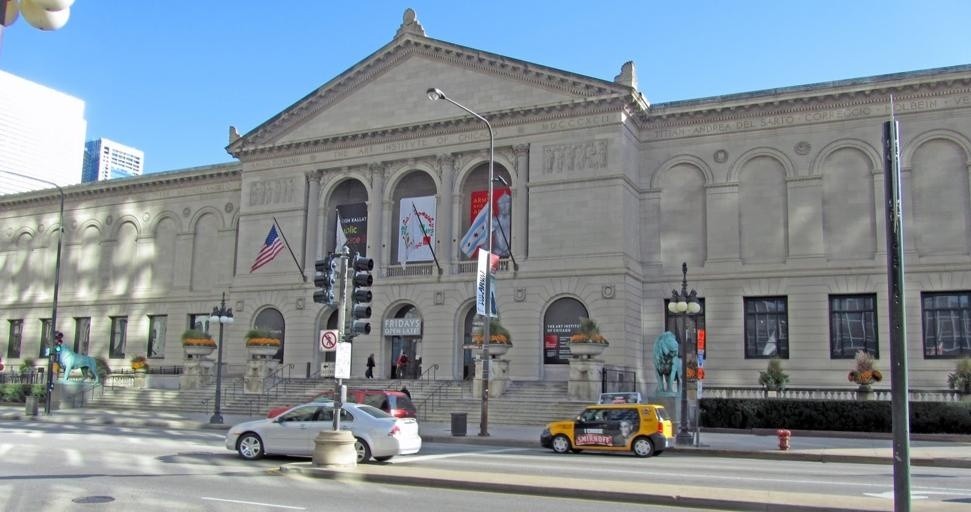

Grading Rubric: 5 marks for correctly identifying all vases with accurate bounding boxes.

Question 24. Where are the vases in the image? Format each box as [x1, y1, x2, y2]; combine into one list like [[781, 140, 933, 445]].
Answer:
[[475, 343, 512, 360], [247, 343, 279, 357], [569, 340, 606, 357], [851, 384, 880, 404], [182, 344, 220, 357]]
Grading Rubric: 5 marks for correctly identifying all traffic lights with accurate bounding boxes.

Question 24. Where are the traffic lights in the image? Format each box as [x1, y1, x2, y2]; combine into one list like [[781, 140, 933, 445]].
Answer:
[[311, 257, 330, 303], [354, 257, 376, 337], [51, 332, 63, 353]]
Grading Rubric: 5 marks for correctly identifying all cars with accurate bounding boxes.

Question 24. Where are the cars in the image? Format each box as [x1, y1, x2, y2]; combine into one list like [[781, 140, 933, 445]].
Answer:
[[224, 402, 423, 463]]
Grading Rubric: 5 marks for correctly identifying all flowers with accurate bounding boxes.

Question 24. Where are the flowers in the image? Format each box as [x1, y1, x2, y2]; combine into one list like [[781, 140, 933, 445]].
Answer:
[[843, 349, 882, 387], [468, 321, 511, 346], [562, 313, 609, 343], [242, 329, 285, 345], [177, 321, 220, 343]]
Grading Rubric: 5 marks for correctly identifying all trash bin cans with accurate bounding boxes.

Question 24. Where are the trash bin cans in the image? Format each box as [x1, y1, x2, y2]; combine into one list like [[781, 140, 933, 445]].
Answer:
[[449, 413, 467, 436], [24, 396, 38, 416]]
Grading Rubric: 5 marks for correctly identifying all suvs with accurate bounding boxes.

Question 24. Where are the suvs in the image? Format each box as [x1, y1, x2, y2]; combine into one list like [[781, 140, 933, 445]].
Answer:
[[540, 392, 677, 457], [268, 389, 417, 422]]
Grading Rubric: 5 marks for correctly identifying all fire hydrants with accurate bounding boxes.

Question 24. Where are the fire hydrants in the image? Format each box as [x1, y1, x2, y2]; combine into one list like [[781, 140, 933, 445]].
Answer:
[[778, 429, 793, 451]]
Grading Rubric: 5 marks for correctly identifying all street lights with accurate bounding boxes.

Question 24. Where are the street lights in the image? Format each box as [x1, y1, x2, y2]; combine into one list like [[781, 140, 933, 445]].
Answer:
[[669, 262, 703, 447], [209, 290, 233, 423], [425, 87, 495, 437]]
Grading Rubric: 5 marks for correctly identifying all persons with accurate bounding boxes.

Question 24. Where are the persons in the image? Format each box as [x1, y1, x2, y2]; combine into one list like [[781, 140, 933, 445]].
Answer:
[[367, 354, 376, 378], [397, 351, 411, 378]]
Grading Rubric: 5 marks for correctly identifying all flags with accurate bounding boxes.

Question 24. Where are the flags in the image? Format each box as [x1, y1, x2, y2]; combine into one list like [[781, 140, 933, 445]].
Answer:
[[459, 202, 498, 259], [249, 222, 285, 275], [334, 215, 349, 256], [400, 204, 426, 271]]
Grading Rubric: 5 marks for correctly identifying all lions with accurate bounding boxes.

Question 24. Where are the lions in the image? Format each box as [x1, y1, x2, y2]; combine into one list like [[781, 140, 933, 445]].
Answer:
[[652, 331, 683, 393], [55, 344, 100, 384]]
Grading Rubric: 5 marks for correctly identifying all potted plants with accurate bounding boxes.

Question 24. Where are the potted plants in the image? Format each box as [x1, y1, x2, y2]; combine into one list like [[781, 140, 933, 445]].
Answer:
[[941, 358, 971, 402]]
